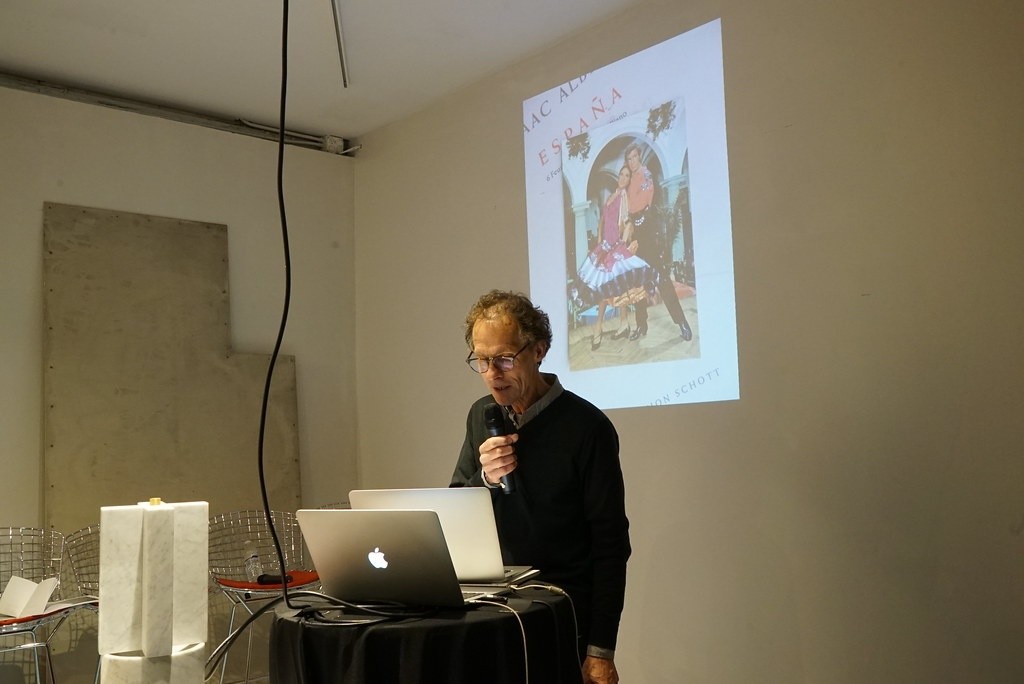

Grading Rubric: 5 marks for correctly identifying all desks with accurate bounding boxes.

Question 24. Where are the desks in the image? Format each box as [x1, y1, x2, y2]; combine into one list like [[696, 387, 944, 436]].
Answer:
[[269, 580, 579, 684]]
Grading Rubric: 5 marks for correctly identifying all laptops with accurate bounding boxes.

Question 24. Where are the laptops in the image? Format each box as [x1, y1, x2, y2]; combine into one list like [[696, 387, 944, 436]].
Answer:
[[348, 487, 534, 584], [296, 508, 518, 614]]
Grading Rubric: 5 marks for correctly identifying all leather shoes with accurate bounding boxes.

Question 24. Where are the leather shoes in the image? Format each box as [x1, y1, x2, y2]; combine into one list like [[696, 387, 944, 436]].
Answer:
[[630, 323, 648, 340], [679, 320, 692, 341]]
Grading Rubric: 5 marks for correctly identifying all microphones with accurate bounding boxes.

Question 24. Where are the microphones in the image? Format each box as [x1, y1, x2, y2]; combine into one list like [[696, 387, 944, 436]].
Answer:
[[257, 574, 293, 585], [482, 403, 515, 496]]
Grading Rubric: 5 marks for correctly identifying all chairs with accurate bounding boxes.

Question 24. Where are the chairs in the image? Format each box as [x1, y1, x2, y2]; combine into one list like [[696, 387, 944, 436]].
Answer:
[[0, 526, 70, 684], [209, 509, 321, 684], [65, 523, 102, 684]]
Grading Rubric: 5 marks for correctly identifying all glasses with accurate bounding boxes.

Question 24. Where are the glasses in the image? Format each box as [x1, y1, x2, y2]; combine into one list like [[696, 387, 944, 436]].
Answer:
[[466, 339, 533, 373]]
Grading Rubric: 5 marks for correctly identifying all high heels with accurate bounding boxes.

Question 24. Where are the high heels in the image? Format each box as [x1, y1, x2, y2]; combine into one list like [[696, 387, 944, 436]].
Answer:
[[611, 328, 630, 340], [591, 331, 602, 351]]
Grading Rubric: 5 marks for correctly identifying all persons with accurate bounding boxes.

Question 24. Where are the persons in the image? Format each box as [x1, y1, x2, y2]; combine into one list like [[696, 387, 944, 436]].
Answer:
[[577, 165, 660, 352], [448, 289, 632, 684], [625, 143, 693, 341]]
